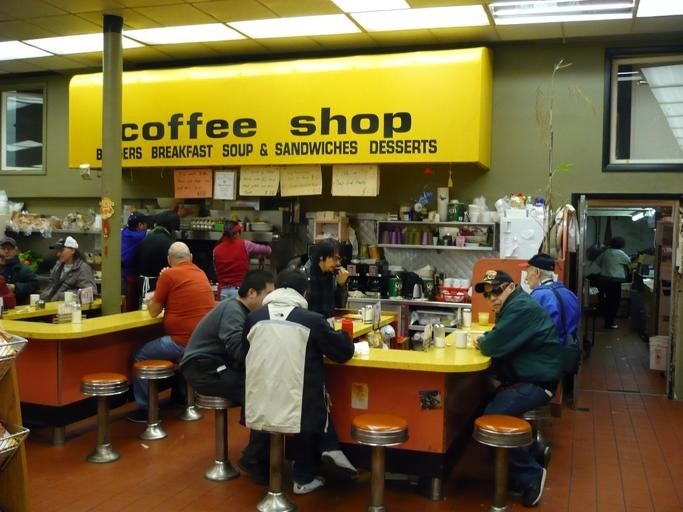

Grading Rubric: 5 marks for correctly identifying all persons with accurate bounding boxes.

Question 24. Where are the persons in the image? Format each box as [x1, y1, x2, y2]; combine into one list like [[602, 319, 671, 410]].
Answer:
[[596, 236, 632, 329], [2, 212, 96, 307], [180, 239, 355, 494], [474, 254, 580, 506], [127, 242, 216, 423], [120, 214, 271, 301]]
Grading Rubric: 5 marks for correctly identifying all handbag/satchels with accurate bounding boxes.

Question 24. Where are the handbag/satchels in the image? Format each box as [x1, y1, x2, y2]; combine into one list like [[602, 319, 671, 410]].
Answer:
[[565, 335, 581, 374]]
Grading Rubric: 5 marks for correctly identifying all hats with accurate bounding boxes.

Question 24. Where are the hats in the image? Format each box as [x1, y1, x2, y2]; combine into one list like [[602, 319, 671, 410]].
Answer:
[[128, 212, 153, 225], [49, 235, 79, 250], [474, 269, 514, 292], [0, 237, 16, 247], [518, 253, 555, 271]]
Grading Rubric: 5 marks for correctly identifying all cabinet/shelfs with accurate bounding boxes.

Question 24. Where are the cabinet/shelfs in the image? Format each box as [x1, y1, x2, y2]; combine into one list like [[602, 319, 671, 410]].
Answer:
[[376, 220, 499, 251], [313, 217, 350, 244]]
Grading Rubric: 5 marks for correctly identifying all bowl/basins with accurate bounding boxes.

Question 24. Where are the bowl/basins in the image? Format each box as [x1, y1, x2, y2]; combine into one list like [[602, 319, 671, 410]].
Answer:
[[157, 198, 184, 209], [444, 292, 466, 303]]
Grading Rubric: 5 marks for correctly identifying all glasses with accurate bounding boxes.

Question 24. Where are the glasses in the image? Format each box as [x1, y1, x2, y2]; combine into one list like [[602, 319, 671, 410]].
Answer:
[[483, 283, 511, 299]]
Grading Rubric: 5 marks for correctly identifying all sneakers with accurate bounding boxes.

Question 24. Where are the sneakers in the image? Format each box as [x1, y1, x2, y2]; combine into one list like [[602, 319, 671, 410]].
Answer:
[[534, 441, 551, 467], [159, 400, 184, 409], [124, 408, 164, 423], [293, 475, 326, 494], [522, 467, 547, 507], [605, 322, 619, 329], [321, 450, 360, 482], [237, 454, 269, 485]]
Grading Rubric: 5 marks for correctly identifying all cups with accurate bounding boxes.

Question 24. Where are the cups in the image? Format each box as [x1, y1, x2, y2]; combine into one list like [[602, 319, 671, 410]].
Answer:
[[463, 308, 472, 326], [478, 312, 490, 326], [469, 204, 496, 222], [381, 230, 438, 245], [454, 331, 479, 349], [357, 304, 372, 325]]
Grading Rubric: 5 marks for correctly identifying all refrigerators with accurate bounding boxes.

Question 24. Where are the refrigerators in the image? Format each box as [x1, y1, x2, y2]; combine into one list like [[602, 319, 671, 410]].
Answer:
[[500, 216, 545, 259]]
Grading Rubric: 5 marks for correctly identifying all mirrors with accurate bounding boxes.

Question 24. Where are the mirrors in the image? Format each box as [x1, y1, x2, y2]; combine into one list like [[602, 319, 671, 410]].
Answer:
[[601, 45, 683, 172], [0, 81, 48, 176]]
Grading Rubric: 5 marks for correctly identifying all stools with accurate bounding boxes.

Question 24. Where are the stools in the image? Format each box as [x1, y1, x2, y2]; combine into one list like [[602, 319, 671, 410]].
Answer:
[[473, 413, 534, 512], [350, 413, 410, 512], [254, 428, 298, 512], [518, 407, 547, 471]]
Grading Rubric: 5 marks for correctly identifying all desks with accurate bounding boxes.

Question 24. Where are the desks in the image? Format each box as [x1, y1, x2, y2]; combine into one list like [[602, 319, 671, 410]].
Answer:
[[0, 295, 497, 502]]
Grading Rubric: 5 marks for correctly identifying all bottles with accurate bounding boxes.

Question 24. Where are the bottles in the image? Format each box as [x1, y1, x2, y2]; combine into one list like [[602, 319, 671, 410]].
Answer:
[[404, 211, 411, 221], [412, 283, 421, 298], [525, 195, 545, 218], [72, 304, 82, 324], [192, 217, 225, 232], [433, 323, 446, 347], [244, 219, 251, 232], [342, 318, 353, 340], [35, 299, 45, 309]]
